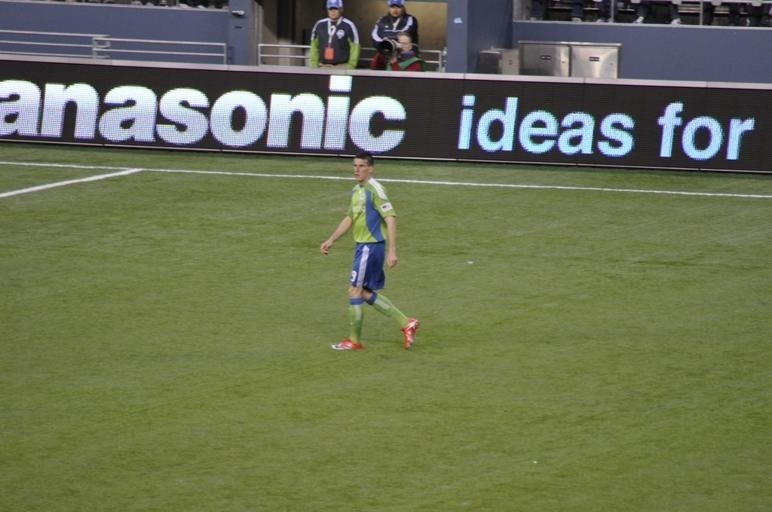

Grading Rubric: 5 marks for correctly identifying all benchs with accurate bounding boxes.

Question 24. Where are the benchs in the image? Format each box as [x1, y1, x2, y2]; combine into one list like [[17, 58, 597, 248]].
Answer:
[[546, 0, 772, 27]]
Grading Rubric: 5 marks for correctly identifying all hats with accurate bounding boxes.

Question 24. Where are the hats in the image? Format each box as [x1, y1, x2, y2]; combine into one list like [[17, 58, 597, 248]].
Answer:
[[326, 0, 343, 9], [387, 0, 405, 7]]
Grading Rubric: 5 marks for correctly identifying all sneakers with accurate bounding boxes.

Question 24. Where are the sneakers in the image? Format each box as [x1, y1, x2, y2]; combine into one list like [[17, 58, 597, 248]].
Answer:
[[401, 318, 419, 349], [331, 339, 362, 350]]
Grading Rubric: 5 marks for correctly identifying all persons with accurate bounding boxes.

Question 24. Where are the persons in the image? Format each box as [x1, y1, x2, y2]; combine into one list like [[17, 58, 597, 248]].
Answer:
[[371, 0, 419, 54], [321, 151, 419, 350], [370, 33, 424, 72], [307, 0, 361, 70], [526, 2, 770, 28]]
